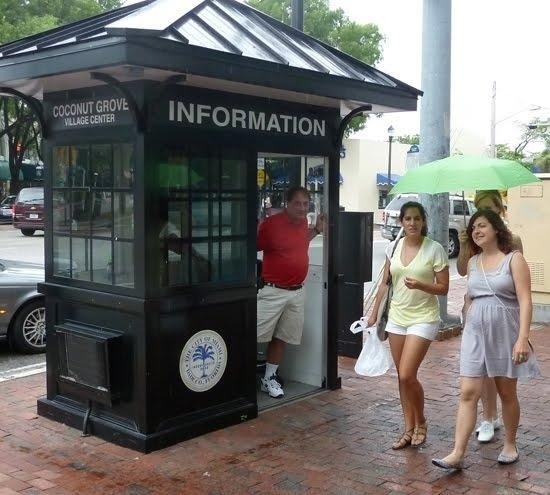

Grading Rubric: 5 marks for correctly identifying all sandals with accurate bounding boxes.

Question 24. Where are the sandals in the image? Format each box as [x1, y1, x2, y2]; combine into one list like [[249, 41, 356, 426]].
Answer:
[[409, 419, 428, 449], [390, 430, 414, 452]]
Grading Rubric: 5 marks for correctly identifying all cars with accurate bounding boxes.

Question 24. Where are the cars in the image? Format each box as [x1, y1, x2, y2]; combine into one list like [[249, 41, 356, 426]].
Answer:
[[1, 193, 17, 219], [0, 256, 81, 353]]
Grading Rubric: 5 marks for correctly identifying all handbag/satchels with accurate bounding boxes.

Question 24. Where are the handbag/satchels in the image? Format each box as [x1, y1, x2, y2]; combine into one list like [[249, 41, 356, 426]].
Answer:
[[375, 308, 392, 342]]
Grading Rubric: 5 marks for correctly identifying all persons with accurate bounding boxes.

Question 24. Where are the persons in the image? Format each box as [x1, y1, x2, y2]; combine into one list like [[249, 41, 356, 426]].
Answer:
[[366, 202, 449, 449], [430, 210, 532, 470], [457, 189, 524, 443], [262, 192, 273, 217], [256, 187, 324, 399]]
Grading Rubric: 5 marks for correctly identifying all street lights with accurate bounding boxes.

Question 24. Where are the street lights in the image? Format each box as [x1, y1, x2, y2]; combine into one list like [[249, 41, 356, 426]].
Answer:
[[385, 124, 396, 197]]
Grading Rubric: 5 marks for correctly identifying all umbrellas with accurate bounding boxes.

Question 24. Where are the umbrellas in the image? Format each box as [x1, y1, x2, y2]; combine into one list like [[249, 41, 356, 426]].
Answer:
[[388, 153, 542, 228], [159, 163, 203, 187]]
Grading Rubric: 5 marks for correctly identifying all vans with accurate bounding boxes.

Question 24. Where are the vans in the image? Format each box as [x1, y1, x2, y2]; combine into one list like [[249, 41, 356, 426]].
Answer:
[[12, 187, 72, 238]]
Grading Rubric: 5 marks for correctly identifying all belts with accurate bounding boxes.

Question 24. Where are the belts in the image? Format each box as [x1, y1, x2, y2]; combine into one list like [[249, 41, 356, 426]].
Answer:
[[262, 281, 305, 291]]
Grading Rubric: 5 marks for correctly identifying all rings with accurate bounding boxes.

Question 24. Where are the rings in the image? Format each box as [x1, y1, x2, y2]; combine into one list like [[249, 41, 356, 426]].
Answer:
[[518, 354, 524, 361]]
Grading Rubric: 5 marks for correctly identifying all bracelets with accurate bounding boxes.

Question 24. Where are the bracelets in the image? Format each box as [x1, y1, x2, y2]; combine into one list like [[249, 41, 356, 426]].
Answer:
[[314, 228, 320, 235]]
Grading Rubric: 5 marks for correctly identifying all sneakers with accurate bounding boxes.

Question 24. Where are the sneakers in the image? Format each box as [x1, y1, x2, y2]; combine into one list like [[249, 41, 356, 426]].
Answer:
[[430, 453, 465, 473], [259, 377, 285, 400], [474, 418, 503, 435], [496, 444, 520, 466], [476, 420, 496, 445]]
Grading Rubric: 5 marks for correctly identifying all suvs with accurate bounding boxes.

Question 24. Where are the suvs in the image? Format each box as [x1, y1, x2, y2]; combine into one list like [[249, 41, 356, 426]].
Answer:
[[381, 192, 482, 258]]
